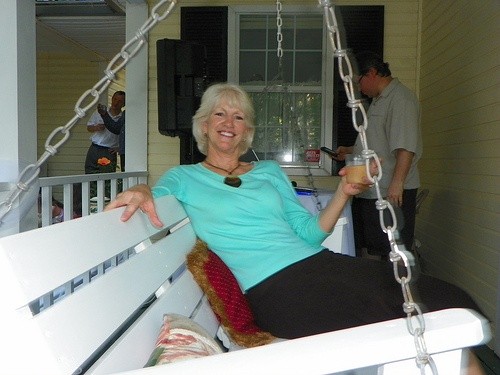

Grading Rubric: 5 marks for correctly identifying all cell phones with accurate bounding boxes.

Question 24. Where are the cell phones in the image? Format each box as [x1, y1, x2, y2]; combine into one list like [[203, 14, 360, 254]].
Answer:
[[320, 146, 337, 157], [101, 105, 106, 112]]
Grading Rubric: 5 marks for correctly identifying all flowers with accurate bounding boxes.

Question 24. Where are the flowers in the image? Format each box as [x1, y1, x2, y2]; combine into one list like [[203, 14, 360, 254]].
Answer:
[[90, 156, 120, 197]]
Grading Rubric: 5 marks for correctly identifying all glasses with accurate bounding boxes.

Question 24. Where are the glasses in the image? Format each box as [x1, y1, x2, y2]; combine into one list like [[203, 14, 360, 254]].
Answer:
[[353, 70, 370, 88]]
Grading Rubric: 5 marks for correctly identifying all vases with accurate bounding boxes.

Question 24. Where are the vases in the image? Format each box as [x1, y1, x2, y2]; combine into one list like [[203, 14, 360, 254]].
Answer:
[[90, 197, 110, 213]]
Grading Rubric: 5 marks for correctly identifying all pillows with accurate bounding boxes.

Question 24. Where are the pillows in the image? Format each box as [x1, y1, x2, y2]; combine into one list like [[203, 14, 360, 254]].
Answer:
[[148, 314, 226, 366], [186, 239, 271, 349]]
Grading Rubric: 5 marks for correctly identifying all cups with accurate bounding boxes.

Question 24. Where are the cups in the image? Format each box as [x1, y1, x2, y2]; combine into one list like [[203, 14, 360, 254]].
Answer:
[[344, 153, 372, 184]]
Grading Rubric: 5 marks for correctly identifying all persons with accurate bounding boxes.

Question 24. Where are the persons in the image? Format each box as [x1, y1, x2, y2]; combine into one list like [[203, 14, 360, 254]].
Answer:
[[84, 90, 125, 174], [103, 83, 492, 374], [329, 51, 424, 266], [97, 103, 125, 172]]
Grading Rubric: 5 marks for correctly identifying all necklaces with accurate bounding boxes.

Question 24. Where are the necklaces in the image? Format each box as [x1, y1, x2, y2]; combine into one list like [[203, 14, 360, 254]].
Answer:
[[199, 153, 254, 189]]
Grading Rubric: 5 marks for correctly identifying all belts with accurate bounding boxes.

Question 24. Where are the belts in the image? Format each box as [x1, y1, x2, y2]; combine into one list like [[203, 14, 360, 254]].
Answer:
[[92, 143, 109, 150]]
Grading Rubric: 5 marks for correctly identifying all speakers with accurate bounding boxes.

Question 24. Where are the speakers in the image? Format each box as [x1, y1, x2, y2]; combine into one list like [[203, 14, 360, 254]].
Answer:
[[156, 38, 207, 138]]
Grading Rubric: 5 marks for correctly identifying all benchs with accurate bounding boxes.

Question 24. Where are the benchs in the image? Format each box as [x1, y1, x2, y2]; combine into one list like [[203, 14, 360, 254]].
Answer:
[[0, 194, 494, 375]]
